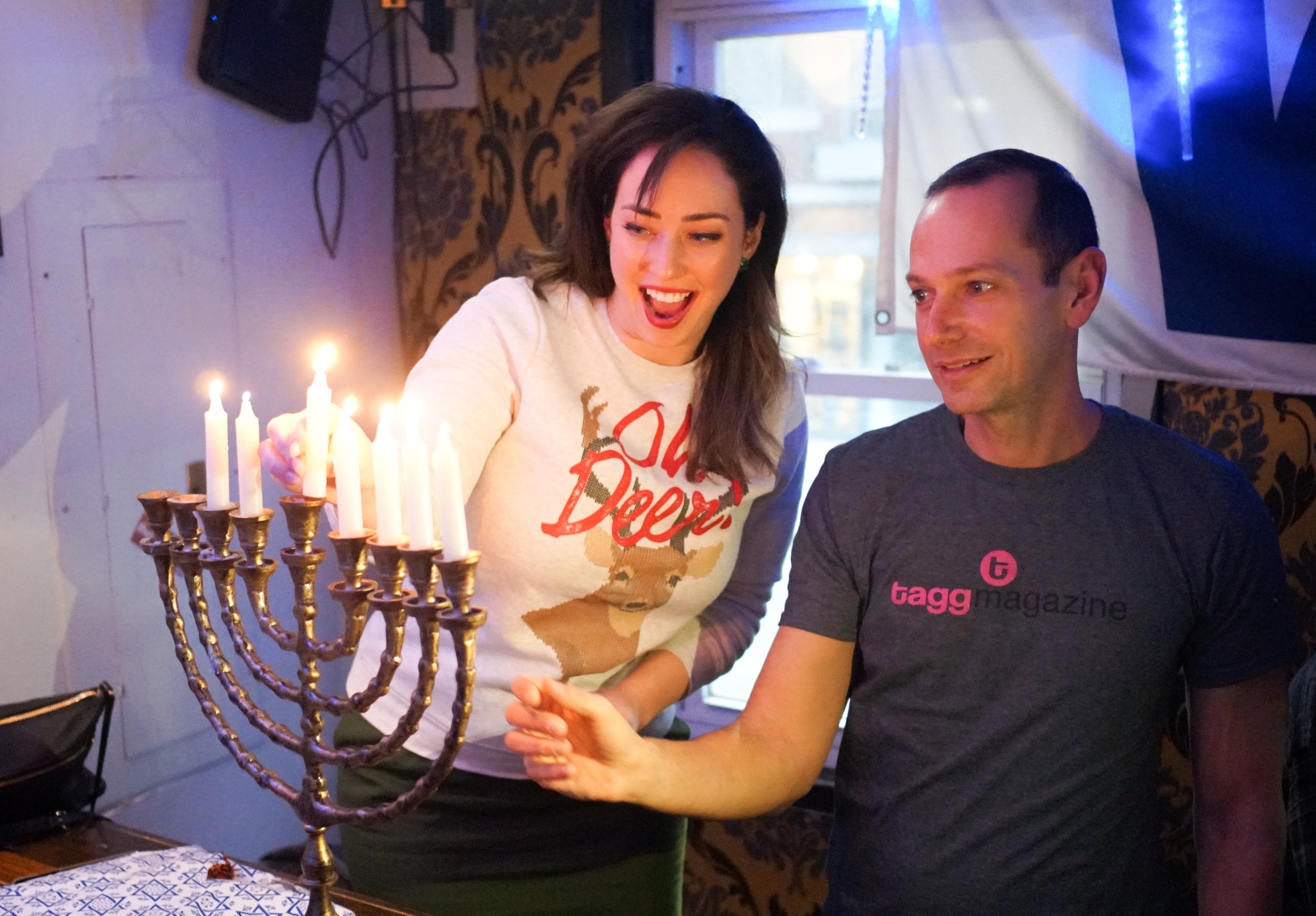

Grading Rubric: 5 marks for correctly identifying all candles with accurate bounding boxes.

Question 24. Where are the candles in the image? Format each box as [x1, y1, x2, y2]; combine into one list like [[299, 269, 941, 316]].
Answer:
[[203, 337, 469, 563]]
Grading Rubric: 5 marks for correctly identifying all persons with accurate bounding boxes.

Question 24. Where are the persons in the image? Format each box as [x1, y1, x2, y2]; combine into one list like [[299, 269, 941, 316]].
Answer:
[[505, 148, 1286, 916], [257, 84, 808, 915]]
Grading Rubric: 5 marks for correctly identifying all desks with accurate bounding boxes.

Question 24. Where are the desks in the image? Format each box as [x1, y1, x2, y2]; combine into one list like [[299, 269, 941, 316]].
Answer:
[[1, 813, 435, 916]]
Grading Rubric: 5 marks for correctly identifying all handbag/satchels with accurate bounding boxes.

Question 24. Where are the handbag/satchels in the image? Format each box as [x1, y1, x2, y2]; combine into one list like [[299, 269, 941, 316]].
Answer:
[[0, 681, 115, 851]]
